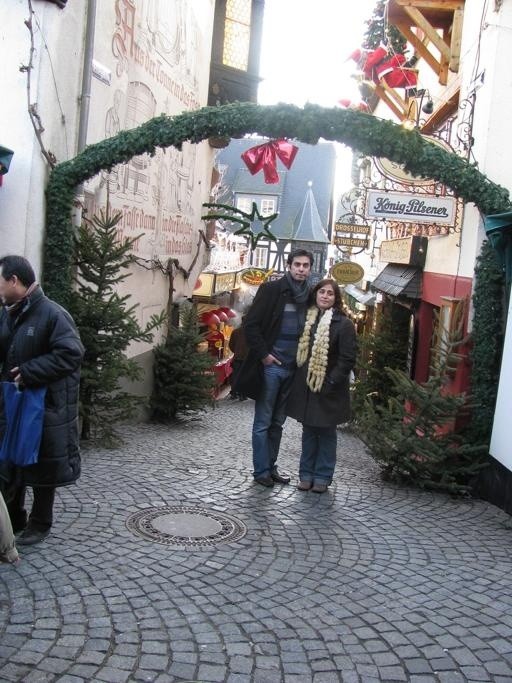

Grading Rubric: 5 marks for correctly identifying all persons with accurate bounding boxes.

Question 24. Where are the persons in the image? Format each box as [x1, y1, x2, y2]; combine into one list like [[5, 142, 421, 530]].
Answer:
[[244, 247, 315, 487], [283, 279, 356, 493], [229, 313, 251, 400], [1, 254, 86, 544], [0, 489, 19, 563]]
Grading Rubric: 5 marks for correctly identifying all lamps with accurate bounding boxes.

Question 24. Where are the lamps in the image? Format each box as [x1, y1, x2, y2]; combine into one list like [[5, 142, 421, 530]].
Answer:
[[404, 87, 434, 131]]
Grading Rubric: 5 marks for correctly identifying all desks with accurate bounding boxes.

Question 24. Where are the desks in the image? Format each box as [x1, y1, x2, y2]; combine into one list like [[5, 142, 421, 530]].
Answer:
[[209, 352, 235, 385]]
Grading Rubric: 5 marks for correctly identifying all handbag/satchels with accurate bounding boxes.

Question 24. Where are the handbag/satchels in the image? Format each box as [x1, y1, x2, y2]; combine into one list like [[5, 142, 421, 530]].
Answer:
[[1, 380, 47, 465]]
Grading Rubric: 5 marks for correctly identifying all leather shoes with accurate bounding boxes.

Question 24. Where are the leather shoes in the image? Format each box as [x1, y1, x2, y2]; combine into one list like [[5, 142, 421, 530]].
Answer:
[[15, 525, 52, 545], [255, 469, 328, 495]]
